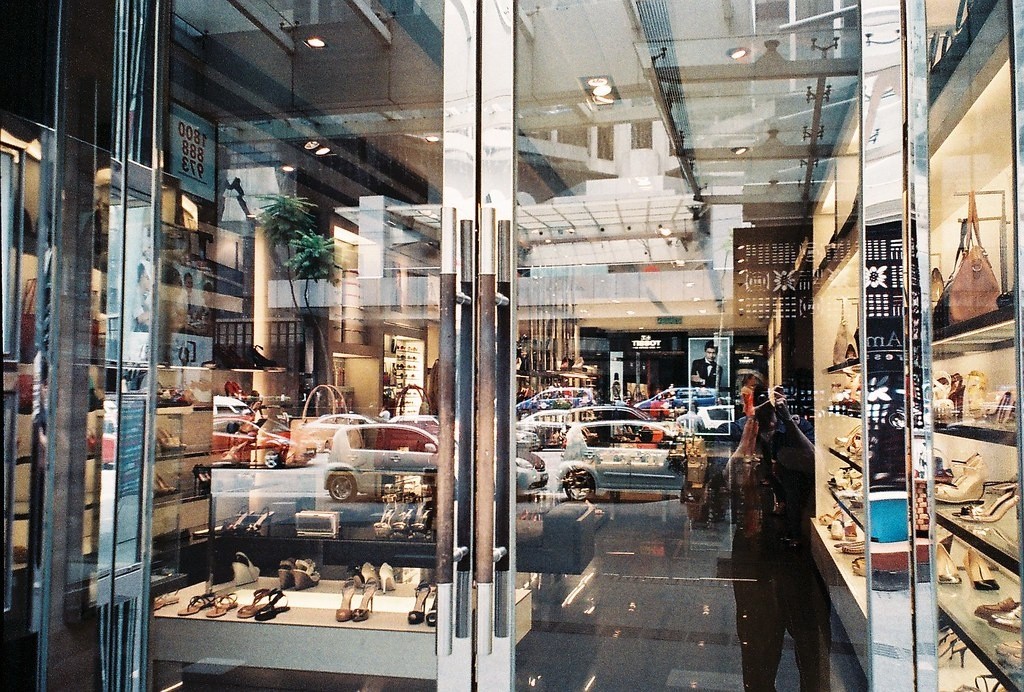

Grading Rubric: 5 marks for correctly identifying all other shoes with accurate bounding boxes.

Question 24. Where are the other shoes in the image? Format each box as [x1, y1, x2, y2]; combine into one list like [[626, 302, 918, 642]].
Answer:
[[214, 344, 277, 369]]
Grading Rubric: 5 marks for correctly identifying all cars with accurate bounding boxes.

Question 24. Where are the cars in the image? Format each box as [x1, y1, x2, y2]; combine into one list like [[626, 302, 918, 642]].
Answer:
[[210, 413, 313, 470], [323, 423, 547, 504], [316, 384, 747, 445], [561, 419, 695, 506]]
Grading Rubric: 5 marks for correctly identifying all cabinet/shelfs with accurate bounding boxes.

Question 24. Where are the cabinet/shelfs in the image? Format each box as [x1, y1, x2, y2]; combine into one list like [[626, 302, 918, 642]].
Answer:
[[805, 3, 1024, 692], [7, 136, 248, 616]]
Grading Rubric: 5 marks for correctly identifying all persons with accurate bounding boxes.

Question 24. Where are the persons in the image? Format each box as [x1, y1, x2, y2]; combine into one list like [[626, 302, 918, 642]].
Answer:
[[677, 405, 708, 433], [691, 339, 724, 405], [713, 360, 833, 692]]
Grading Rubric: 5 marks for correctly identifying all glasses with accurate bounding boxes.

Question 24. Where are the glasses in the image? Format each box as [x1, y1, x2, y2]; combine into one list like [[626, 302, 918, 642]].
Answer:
[[142, 275, 150, 280], [393, 346, 417, 416]]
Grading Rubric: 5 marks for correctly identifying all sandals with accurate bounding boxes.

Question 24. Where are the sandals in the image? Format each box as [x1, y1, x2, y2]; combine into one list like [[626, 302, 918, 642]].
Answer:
[[155, 589, 290, 621]]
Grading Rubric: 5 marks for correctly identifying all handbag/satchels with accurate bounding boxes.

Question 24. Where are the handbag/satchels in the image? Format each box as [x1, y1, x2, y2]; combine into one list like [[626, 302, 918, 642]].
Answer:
[[833, 191, 1000, 367], [20, 278, 38, 363], [382, 362, 389, 387], [19, 374, 33, 413], [286, 385, 363, 467], [374, 386, 440, 466]]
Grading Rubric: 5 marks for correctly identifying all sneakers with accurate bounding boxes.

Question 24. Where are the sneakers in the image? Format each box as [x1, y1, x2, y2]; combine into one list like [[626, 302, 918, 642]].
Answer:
[[743, 454, 761, 464]]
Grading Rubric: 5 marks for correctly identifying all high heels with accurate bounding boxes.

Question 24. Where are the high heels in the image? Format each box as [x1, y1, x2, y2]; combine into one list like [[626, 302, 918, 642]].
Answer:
[[221, 505, 274, 536], [278, 558, 321, 591], [154, 473, 179, 495], [819, 374, 1024, 692], [193, 464, 211, 488], [374, 502, 433, 539], [220, 382, 262, 462], [233, 552, 260, 586], [157, 427, 187, 450], [519, 510, 541, 521], [336, 562, 438, 628]]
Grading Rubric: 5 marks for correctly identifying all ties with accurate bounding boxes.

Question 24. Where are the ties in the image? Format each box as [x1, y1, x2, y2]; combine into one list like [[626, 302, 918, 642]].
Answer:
[[188, 295, 192, 310]]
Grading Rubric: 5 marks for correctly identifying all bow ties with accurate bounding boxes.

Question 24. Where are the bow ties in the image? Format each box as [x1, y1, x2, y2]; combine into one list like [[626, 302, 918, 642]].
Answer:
[[705, 363, 715, 367]]
